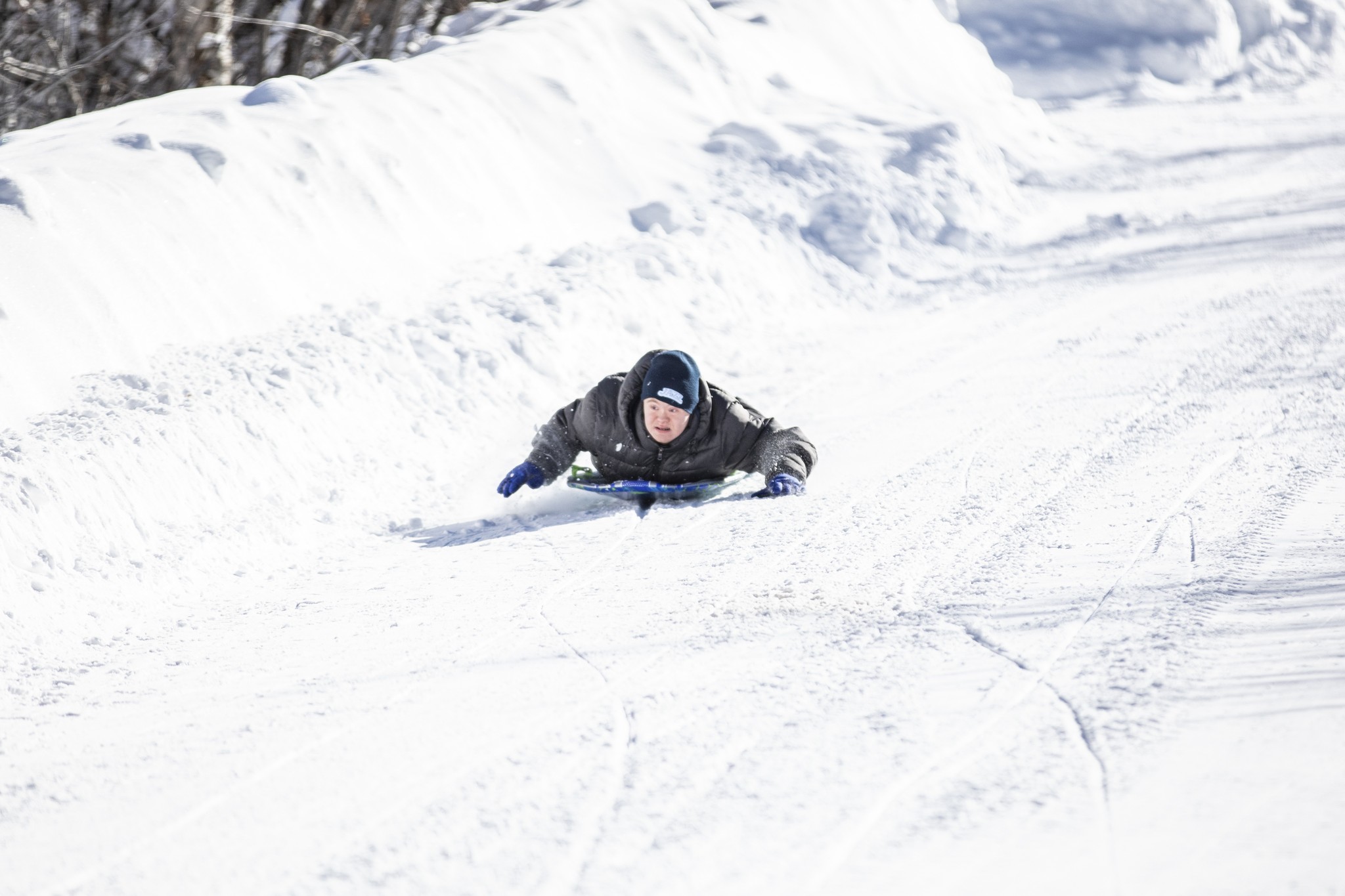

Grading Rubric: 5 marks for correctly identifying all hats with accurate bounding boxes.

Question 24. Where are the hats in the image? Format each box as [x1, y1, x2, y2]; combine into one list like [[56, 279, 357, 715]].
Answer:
[[641, 350, 701, 414]]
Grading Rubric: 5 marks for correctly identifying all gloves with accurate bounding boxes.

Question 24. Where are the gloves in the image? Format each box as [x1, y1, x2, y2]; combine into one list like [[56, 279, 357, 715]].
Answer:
[[751, 473, 807, 497], [496, 462, 544, 497]]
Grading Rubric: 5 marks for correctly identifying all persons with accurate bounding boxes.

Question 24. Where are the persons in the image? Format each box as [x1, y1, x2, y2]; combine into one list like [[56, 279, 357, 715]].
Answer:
[[496, 348, 820, 500]]
